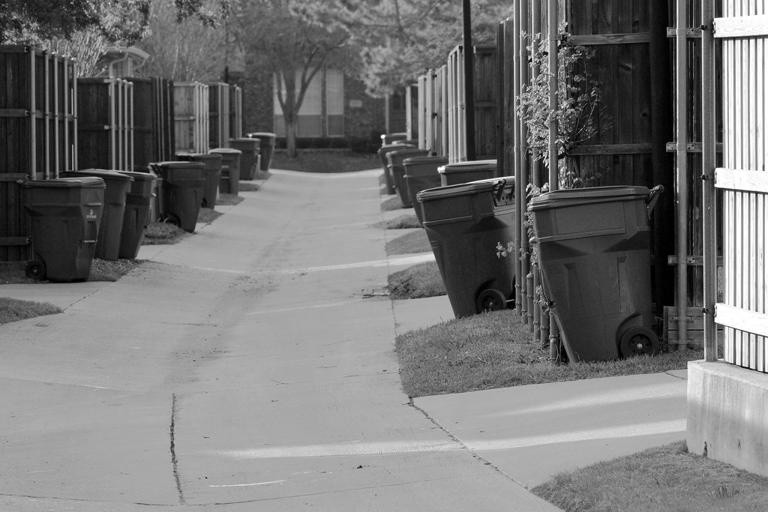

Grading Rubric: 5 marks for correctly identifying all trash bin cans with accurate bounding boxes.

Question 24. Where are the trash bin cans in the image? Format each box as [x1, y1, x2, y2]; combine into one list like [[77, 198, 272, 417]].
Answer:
[[377, 132, 498, 228], [526, 184, 665, 366], [22, 132, 276, 282], [416, 176, 515, 318]]
